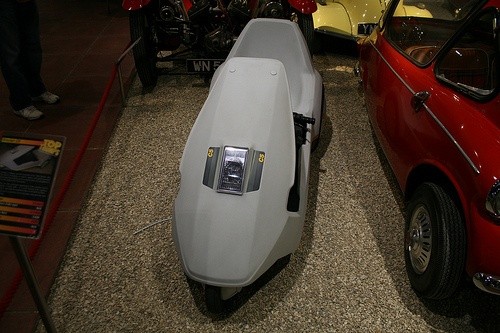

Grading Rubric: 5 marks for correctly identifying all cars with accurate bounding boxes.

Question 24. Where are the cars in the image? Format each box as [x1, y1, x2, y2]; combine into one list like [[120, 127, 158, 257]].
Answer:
[[354, 0, 500, 301], [121, 1, 317, 89]]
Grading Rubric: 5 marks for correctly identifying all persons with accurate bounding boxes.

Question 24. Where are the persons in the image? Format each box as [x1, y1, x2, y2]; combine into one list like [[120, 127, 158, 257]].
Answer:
[[0, 0, 60, 121]]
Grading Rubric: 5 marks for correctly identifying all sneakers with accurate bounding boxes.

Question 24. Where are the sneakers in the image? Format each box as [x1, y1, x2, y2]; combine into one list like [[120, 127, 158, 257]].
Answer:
[[31, 91, 59, 105], [13, 105, 44, 121]]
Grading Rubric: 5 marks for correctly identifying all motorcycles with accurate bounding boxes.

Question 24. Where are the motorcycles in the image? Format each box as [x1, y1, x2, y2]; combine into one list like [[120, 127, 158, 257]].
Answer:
[[172, 18, 326, 315]]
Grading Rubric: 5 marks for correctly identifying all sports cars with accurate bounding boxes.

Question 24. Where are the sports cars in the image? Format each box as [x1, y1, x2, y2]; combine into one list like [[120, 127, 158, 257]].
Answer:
[[313, 0, 433, 51]]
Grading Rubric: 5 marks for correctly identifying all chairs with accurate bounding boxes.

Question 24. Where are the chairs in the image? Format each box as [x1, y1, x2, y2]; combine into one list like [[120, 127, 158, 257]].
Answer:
[[406, 45, 491, 91]]
